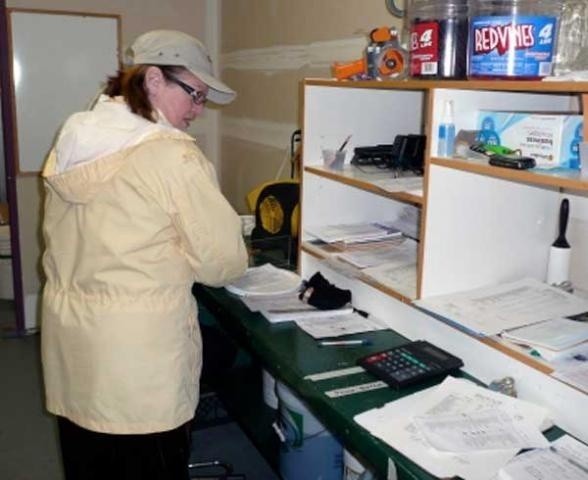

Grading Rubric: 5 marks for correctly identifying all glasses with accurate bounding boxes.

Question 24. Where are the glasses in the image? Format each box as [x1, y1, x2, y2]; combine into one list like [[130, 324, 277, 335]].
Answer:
[[162, 70, 207, 105]]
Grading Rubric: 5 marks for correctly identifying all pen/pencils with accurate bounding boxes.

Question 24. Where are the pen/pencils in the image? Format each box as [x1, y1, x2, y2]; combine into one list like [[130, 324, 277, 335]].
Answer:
[[318, 339, 372, 347], [338, 134, 353, 153]]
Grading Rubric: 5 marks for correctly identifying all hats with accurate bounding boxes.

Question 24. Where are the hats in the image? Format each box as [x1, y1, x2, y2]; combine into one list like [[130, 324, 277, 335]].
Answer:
[[122, 29, 238, 106]]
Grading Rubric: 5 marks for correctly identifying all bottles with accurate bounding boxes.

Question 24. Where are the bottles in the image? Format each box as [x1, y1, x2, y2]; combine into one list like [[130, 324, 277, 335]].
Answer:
[[404, 0, 468, 80], [438, 100, 456, 157]]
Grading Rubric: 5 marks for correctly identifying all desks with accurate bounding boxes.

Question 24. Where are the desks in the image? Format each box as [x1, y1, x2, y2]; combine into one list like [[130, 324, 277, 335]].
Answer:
[[194, 271, 588, 480]]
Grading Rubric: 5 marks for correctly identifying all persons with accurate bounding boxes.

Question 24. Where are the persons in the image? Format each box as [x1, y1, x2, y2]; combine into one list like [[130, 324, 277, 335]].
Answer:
[[41, 29, 248, 480]]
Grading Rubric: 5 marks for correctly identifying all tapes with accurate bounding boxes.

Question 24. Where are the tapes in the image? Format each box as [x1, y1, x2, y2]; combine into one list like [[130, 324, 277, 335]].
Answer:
[[374, 44, 409, 82]]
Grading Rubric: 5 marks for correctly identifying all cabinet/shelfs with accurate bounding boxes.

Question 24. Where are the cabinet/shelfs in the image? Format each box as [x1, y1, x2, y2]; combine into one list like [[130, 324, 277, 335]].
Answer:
[[295, 74, 587, 434]]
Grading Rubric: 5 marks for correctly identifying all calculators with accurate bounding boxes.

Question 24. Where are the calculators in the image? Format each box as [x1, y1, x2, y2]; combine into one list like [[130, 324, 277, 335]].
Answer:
[[356, 339, 465, 392]]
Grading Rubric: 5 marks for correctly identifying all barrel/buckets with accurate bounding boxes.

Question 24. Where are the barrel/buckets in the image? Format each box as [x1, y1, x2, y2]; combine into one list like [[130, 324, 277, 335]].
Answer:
[[271, 381, 344, 480]]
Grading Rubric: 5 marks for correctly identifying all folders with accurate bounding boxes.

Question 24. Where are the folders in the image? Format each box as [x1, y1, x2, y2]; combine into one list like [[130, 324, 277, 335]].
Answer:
[[414, 275, 588, 340]]
